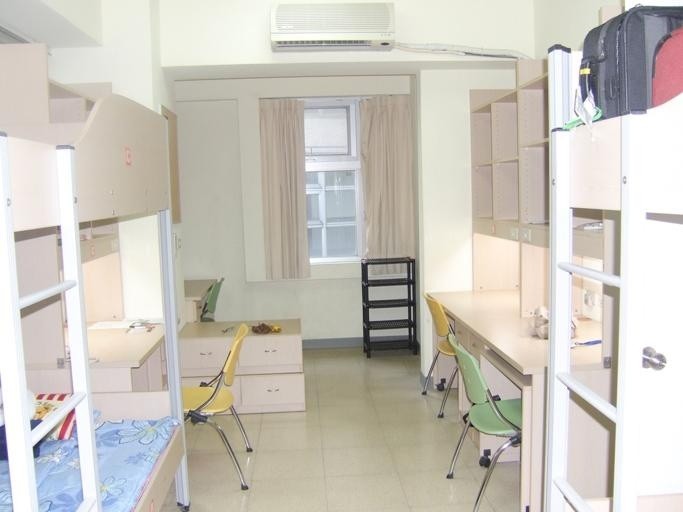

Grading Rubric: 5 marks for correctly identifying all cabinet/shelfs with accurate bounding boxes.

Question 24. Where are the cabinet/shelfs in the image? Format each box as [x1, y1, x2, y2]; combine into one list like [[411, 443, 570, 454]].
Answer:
[[362, 257, 418, 358], [468, 58, 622, 369], [179, 319, 306, 415]]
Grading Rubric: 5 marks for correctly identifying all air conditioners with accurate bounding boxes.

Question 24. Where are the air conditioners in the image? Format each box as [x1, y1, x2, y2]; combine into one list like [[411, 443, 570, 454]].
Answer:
[[270, 3, 395, 52]]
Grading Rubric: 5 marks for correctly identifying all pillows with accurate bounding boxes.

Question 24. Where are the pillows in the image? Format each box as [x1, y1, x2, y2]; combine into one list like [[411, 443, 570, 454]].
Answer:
[[1, 394, 75, 460]]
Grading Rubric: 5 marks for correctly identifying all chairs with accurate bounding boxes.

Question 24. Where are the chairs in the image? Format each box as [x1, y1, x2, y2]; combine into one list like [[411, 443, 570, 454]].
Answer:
[[201, 277, 225, 322], [422, 291, 522, 511], [183, 323, 253, 489]]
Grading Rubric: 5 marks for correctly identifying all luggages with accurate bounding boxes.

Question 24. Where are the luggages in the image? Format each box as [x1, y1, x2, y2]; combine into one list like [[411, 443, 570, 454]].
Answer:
[[577, 7, 683, 121]]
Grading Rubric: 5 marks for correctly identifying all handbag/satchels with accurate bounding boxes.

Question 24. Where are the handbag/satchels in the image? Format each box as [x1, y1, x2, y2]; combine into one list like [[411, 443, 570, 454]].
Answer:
[[534, 308, 575, 339]]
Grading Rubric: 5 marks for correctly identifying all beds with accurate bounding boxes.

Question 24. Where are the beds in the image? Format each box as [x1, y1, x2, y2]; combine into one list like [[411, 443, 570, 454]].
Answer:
[[1, 94, 190, 511]]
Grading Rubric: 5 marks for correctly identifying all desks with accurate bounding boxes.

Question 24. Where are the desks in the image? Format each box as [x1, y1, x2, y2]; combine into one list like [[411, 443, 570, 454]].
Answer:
[[184, 279, 217, 322], [427, 289, 602, 512], [86, 320, 166, 392]]
